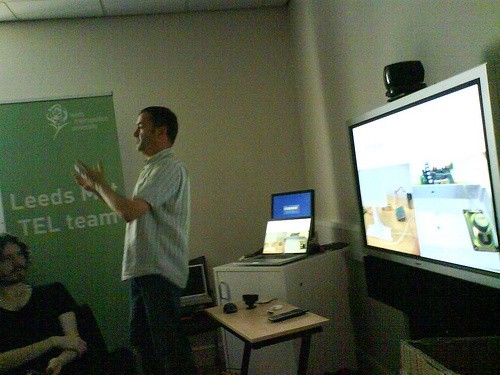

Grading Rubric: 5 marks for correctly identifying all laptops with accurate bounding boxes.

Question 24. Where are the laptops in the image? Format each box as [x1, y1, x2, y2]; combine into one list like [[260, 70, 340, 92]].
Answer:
[[179, 263, 212, 306], [233, 217, 313, 265]]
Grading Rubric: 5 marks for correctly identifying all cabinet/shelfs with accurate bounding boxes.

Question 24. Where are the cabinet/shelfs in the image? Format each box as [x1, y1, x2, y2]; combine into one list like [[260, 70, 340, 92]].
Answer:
[[212, 248, 358, 375]]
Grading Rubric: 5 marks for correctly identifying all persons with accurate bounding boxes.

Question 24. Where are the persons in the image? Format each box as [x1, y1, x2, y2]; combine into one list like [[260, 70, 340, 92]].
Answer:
[[73, 106, 197, 375], [0, 233, 87, 375]]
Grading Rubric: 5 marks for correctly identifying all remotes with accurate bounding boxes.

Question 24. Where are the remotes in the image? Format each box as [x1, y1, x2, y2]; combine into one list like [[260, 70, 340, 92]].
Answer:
[[268, 307, 310, 323]]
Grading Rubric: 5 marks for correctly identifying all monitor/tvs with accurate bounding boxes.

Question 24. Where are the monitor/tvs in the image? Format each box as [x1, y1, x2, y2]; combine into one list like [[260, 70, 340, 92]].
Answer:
[[345, 61, 500, 289], [270, 188, 315, 241]]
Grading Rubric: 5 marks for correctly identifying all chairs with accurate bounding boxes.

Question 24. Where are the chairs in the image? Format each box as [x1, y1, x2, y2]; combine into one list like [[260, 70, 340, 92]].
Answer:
[[178, 255, 220, 370], [55, 280, 107, 358]]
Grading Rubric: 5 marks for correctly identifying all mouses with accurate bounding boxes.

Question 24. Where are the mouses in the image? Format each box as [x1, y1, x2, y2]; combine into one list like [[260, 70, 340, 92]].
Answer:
[[223, 303, 237, 314]]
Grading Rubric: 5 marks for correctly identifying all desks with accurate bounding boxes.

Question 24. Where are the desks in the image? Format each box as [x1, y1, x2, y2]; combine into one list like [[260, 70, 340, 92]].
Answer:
[[203, 297, 330, 375]]
